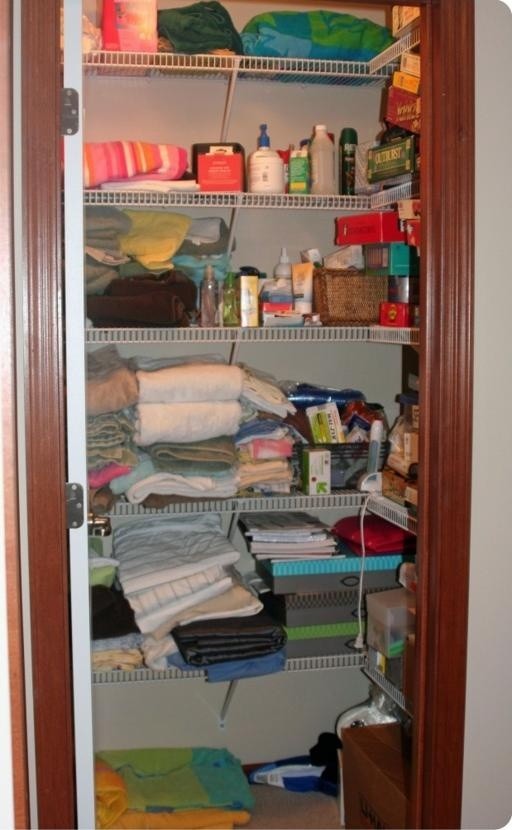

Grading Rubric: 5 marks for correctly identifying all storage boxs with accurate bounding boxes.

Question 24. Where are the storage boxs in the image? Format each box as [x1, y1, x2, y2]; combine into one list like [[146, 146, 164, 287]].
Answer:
[[340, 723, 408, 830]]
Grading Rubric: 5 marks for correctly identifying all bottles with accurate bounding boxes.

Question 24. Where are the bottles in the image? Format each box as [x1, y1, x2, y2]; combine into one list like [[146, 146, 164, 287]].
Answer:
[[274, 247, 291, 276], [201, 263, 218, 324], [310, 124, 336, 194], [223, 272, 240, 325]]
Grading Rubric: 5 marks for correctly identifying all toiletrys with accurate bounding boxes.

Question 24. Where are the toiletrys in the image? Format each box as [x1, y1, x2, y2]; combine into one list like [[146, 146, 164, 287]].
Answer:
[[222, 272, 239, 326], [290, 261, 314, 323], [199, 266, 219, 328]]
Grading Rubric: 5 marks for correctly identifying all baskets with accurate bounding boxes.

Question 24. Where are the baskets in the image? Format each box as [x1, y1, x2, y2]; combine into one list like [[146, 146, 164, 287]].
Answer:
[[314, 270, 388, 325]]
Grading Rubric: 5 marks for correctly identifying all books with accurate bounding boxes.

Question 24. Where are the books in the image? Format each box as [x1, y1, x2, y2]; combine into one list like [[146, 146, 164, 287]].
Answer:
[[239, 511, 346, 562]]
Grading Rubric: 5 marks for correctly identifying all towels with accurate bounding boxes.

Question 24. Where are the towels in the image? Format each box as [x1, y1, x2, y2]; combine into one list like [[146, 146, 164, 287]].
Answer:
[[86, 344, 307, 506], [81, 139, 188, 190], [84, 209, 237, 331]]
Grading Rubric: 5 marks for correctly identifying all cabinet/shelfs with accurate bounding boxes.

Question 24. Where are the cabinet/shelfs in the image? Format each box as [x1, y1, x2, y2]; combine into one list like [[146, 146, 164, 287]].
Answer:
[[63, 3, 419, 830]]
